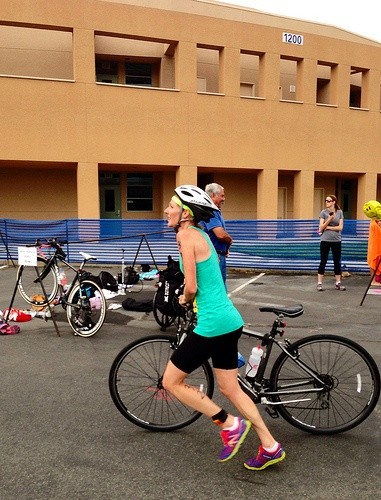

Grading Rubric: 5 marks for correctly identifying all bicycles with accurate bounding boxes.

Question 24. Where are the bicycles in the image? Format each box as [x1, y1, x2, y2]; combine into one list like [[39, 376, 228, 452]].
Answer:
[[16, 237, 106, 337], [110, 281, 381, 435]]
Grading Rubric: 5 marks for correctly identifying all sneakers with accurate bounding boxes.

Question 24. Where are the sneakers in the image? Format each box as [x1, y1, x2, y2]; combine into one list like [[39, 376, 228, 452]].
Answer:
[[244, 442, 286, 470], [217, 418, 252, 463]]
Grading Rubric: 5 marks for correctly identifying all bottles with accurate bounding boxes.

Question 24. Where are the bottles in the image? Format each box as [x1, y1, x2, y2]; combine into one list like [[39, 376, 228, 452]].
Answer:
[[59, 266, 67, 287], [238, 352, 245, 368], [96, 296, 101, 309], [245, 345, 264, 378]]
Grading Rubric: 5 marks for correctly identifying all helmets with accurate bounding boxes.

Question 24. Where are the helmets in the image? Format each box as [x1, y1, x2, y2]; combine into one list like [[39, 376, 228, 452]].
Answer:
[[173, 185, 220, 213]]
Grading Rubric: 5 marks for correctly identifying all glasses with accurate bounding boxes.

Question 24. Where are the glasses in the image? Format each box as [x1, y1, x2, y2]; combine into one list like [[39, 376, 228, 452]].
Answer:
[[325, 199, 335, 203]]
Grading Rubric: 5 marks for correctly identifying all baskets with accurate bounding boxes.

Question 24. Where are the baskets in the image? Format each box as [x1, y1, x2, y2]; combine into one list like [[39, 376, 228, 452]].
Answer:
[[160, 268, 185, 314]]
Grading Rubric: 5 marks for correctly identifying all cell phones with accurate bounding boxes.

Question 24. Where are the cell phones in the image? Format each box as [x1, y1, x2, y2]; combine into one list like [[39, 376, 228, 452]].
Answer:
[[329, 212, 333, 216]]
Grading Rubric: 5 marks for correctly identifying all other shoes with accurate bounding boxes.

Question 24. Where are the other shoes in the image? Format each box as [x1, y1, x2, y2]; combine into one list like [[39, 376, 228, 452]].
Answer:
[[335, 283, 346, 290], [316, 282, 323, 291]]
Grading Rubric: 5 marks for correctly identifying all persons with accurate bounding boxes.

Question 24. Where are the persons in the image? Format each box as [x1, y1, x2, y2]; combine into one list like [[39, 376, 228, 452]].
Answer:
[[161, 185, 286, 471], [198, 182, 233, 284], [315, 194, 346, 291]]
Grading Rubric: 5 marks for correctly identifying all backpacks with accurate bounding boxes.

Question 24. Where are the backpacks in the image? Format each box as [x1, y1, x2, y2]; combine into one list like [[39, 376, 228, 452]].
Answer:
[[124, 267, 140, 284], [99, 271, 118, 292]]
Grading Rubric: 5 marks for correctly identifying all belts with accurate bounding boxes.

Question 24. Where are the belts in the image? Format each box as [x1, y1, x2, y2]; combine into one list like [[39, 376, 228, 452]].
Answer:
[[217, 250, 228, 256]]
